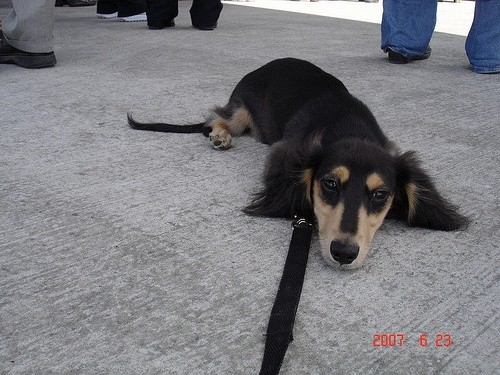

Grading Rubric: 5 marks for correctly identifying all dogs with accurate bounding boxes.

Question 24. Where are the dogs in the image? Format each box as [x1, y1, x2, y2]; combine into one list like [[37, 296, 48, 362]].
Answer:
[[126, 58, 471, 270]]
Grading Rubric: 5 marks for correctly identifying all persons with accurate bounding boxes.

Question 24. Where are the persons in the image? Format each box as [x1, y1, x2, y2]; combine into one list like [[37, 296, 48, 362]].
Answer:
[[0, 0, 57, 69], [381, 0, 500, 74], [55, 0, 223, 31]]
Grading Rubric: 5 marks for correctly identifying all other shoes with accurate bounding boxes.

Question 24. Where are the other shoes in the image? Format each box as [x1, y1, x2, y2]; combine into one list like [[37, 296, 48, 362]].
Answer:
[[388, 47, 432, 64], [0, 36, 57, 68], [96, 12, 147, 22], [55, 0, 96, 7], [146, 17, 218, 31]]
